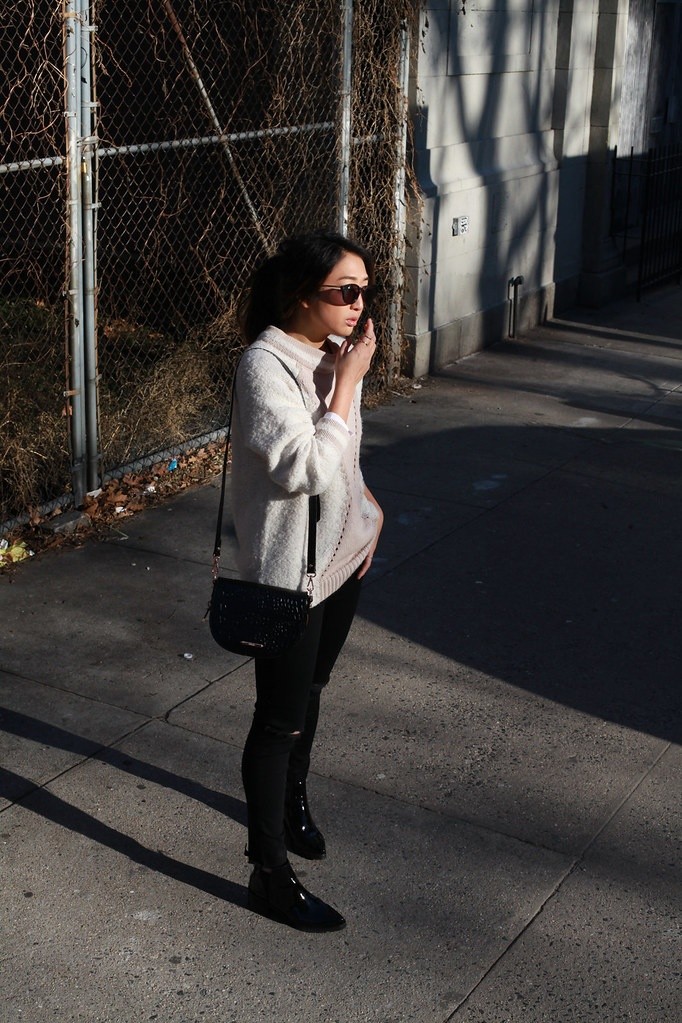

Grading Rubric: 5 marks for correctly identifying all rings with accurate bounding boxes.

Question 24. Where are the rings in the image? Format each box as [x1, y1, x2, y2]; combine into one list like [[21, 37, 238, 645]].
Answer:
[[365, 335, 371, 340], [362, 340, 367, 345]]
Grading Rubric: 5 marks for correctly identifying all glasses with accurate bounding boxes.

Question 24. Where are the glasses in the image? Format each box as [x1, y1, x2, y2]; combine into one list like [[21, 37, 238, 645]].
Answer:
[[322, 284, 377, 305]]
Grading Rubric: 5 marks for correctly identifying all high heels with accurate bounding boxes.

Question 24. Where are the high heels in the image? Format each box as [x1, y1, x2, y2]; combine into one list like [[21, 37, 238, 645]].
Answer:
[[248, 860, 346, 931], [285, 779, 325, 858]]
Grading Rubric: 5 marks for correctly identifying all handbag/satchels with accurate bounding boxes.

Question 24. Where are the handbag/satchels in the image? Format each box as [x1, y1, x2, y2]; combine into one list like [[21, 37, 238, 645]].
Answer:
[[202, 576, 310, 659]]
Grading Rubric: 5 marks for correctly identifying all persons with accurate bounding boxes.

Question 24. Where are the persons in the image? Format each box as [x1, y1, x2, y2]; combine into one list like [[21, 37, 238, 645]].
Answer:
[[228, 229, 384, 933]]
